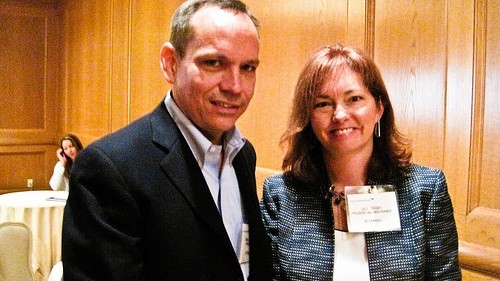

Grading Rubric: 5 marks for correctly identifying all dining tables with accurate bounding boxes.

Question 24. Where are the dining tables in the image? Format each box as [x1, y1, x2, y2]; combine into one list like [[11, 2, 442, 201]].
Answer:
[[0, 190, 69, 281]]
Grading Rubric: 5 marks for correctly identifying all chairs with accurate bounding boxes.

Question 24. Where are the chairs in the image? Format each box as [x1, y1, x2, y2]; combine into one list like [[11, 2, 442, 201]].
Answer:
[[0, 222, 37, 281]]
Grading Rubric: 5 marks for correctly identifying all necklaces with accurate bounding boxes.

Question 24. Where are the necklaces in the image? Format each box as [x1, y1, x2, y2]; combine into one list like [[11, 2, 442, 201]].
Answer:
[[324, 181, 347, 212]]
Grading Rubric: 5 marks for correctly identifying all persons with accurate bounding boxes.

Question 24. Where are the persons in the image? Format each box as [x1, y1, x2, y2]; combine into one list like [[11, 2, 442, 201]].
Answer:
[[48, 134, 84, 191], [260, 42, 463, 281], [59, 0, 276, 281]]
